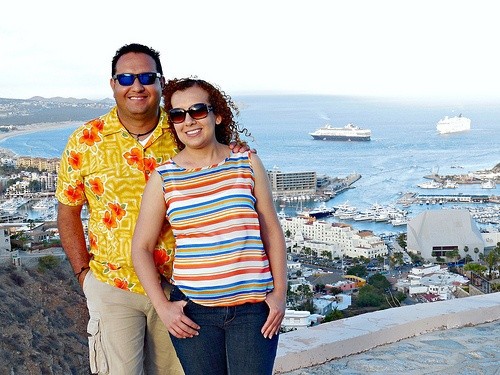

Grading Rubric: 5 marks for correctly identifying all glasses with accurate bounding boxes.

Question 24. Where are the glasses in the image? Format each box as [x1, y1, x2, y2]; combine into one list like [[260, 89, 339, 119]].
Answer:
[[169, 103, 214, 124], [113, 72, 162, 86]]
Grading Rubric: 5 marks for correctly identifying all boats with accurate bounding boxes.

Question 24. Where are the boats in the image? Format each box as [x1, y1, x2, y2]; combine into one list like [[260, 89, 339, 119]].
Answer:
[[417, 178, 497, 189], [437, 113, 470, 134], [311, 123, 372, 142], [0, 196, 54, 213], [309, 200, 410, 227], [468, 204, 500, 225]]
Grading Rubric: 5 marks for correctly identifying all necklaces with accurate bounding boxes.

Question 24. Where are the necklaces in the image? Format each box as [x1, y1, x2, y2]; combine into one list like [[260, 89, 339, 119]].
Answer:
[[117, 106, 162, 142]]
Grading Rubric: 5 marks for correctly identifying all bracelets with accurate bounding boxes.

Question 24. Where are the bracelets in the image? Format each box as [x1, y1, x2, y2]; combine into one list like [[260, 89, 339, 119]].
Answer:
[[70, 266, 91, 280]]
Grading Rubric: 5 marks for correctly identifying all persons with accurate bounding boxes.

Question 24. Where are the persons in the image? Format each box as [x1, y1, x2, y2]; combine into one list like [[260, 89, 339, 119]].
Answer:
[[129, 76, 289, 375], [53, 43, 183, 375]]
